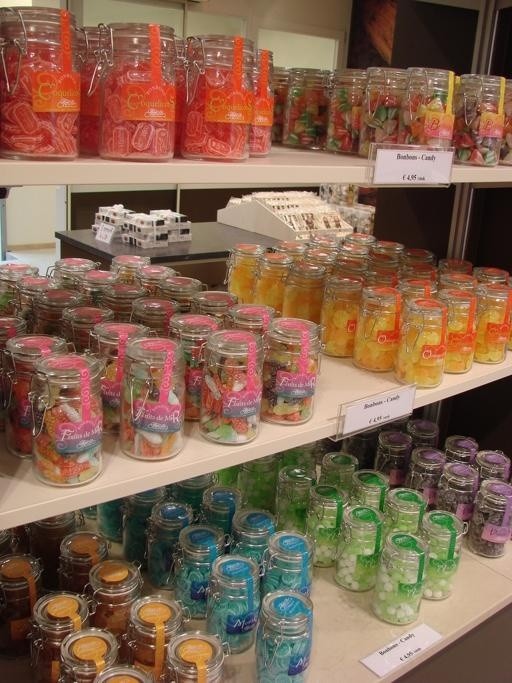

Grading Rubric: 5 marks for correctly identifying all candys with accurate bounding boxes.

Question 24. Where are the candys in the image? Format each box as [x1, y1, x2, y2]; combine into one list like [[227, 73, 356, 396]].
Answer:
[[0, 34, 512, 168], [0, 259, 512, 487], [0, 415, 512, 683]]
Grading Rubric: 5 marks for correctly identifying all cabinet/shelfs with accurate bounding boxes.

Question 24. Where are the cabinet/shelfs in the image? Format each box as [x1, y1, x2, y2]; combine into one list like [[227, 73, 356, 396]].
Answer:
[[0, 0, 512, 682]]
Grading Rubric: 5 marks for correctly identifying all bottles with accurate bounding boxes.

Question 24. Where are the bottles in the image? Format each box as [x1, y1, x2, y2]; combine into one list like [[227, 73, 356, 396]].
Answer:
[[212, 439, 465, 626], [224, 231, 511, 391], [0, 514, 227, 683], [311, 421, 512, 561], [79, 476, 315, 683], [1, 6, 274, 161], [275, 61, 509, 166], [0, 255, 327, 484]]
